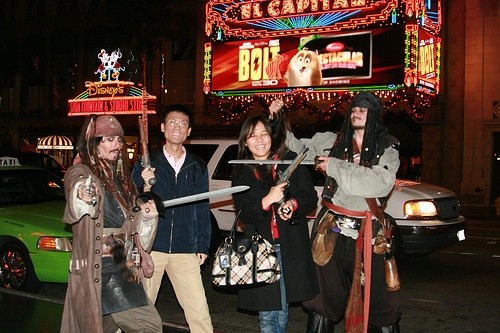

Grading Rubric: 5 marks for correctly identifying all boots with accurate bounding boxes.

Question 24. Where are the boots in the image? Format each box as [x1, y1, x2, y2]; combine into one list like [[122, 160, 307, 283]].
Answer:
[[307, 312, 334, 332], [373, 323, 400, 333]]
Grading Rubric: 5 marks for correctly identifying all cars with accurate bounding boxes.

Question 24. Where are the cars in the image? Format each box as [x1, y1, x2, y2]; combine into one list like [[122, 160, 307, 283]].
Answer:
[[0, 151, 73, 291]]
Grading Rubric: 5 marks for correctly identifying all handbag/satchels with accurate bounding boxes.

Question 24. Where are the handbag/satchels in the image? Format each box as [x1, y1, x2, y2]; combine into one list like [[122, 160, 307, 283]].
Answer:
[[211, 210, 280, 288], [312, 215, 337, 266]]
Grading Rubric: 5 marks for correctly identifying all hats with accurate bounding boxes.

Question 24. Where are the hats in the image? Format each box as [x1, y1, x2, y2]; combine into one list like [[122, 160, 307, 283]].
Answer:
[[92, 114, 125, 138], [351, 91, 382, 115]]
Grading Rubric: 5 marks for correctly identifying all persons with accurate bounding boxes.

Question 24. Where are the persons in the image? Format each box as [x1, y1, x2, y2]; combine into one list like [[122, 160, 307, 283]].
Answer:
[[229, 114, 319, 333], [59, 114, 163, 333], [129, 105, 215, 333], [268, 91, 403, 333]]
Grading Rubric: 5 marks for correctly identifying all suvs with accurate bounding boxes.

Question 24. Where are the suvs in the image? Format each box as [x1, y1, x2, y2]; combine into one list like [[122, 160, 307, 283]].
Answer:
[[183, 137, 465, 255]]
